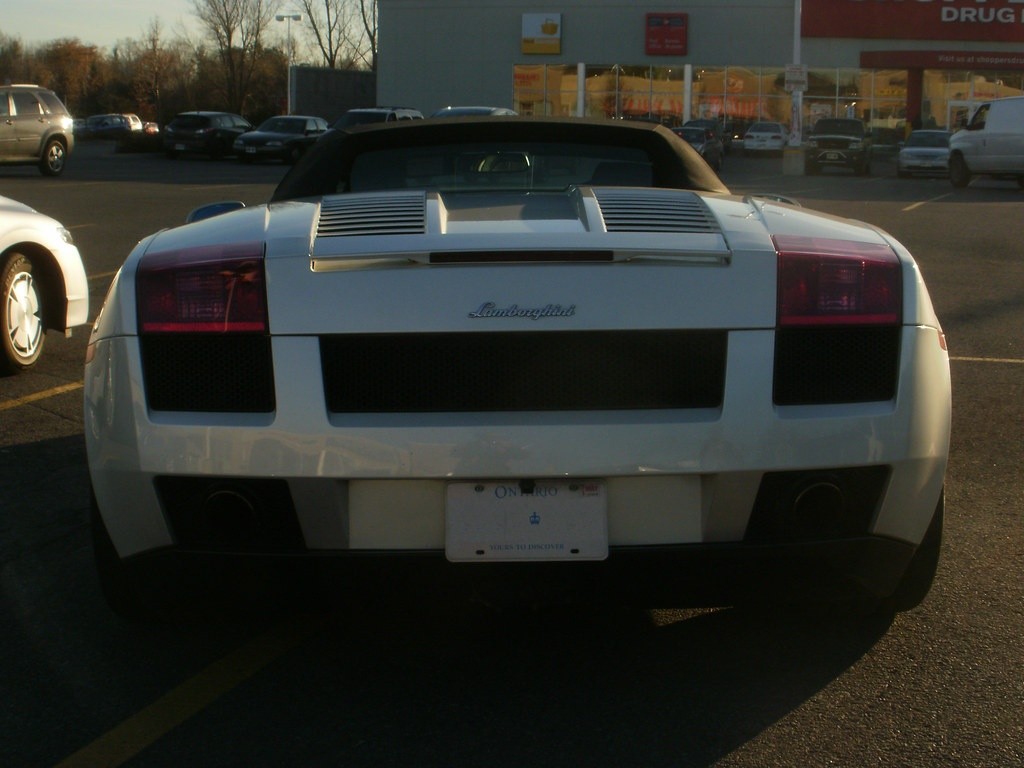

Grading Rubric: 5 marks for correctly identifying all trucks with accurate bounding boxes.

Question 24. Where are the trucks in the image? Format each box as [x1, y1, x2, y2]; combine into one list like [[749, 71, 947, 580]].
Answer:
[[945, 96, 1024, 189]]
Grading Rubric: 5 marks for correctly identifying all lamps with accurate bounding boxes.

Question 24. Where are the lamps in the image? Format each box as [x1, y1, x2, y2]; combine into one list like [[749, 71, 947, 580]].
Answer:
[[693, 73, 705, 82]]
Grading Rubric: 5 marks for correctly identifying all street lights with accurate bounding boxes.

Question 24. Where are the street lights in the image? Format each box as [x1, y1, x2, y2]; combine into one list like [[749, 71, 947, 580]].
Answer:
[[276, 16, 301, 116]]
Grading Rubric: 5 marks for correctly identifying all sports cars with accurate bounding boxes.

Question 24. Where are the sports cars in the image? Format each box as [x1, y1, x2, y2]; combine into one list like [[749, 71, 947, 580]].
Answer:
[[82, 115, 953, 623]]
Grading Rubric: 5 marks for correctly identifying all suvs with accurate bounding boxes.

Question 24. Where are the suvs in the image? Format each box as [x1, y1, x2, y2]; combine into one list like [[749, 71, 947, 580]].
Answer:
[[328, 105, 424, 129], [805, 118, 874, 178]]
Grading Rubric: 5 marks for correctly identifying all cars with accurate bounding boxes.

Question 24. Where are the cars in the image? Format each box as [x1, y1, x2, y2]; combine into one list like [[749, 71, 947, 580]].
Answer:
[[896, 130, 955, 179], [161, 110, 256, 162], [0, 194, 91, 372], [685, 119, 733, 155], [672, 126, 725, 172], [78, 113, 144, 132], [742, 122, 790, 157], [231, 115, 331, 164], [430, 106, 518, 117], [1, 83, 76, 176]]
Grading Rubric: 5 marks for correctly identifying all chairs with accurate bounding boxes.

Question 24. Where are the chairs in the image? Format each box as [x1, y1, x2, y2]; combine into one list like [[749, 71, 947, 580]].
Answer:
[[351, 137, 481, 192], [592, 161, 680, 190]]
